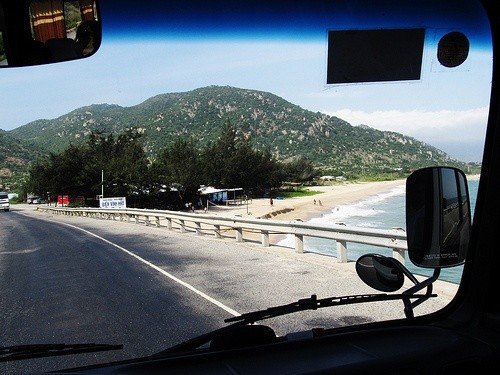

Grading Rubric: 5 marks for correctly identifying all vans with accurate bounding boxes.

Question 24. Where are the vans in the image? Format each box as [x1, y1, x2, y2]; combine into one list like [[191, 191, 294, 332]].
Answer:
[[27, 197, 45, 204], [0, 192, 10, 212]]
